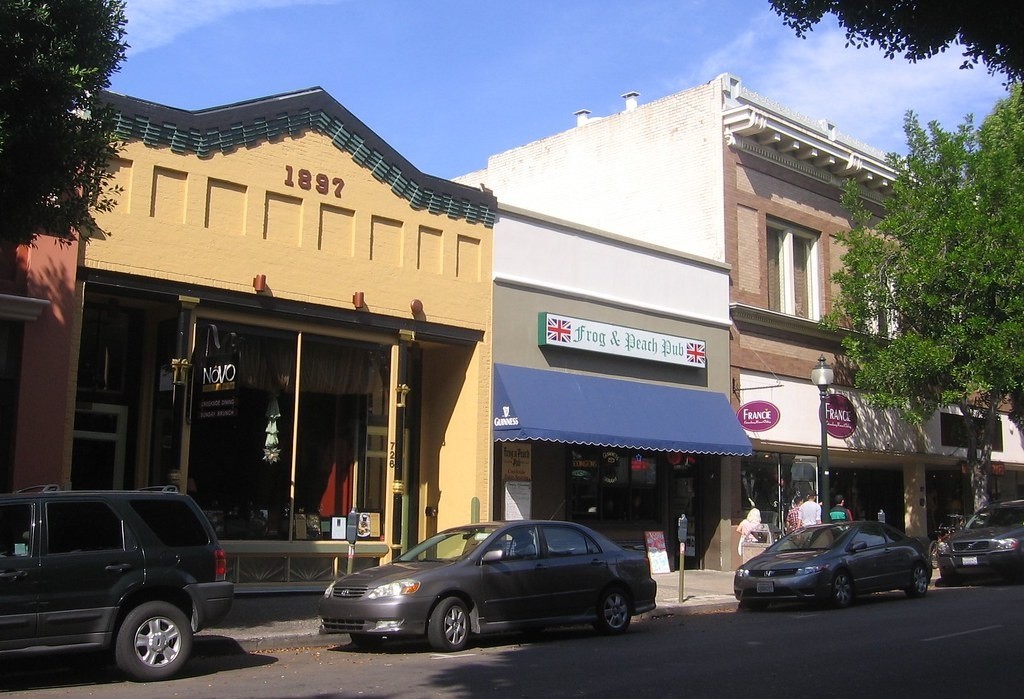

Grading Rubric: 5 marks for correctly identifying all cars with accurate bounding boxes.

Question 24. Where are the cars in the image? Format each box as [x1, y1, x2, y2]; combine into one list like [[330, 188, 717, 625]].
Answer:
[[318, 519, 658, 654], [733, 520, 933, 611]]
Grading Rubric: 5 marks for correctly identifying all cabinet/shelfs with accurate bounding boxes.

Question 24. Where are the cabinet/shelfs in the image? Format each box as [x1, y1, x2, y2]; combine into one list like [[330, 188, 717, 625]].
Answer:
[[74, 401, 129, 488]]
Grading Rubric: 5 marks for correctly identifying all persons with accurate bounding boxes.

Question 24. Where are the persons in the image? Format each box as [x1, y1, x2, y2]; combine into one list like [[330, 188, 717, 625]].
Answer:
[[829, 495, 853, 539], [736, 508, 761, 555], [797, 490, 822, 539], [786, 496, 803, 531]]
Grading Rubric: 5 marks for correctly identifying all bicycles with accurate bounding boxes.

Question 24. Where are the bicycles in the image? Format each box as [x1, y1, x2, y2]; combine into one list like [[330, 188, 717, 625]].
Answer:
[[929, 522, 958, 569]]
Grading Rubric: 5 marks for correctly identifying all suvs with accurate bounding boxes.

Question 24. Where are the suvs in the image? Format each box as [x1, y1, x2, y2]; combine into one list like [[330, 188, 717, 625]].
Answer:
[[0, 484, 235, 681], [937, 497, 1024, 586]]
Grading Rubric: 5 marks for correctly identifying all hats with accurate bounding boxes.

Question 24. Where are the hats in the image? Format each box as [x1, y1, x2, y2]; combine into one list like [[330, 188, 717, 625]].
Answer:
[[833, 494, 845, 504], [806, 490, 817, 496]]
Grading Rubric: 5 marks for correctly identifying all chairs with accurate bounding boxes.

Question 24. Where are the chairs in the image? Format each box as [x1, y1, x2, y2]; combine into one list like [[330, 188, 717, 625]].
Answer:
[[514, 532, 535, 556], [483, 535, 506, 561]]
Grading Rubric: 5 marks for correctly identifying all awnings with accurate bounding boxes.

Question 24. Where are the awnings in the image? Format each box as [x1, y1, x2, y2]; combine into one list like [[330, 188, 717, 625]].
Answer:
[[494, 363, 753, 457]]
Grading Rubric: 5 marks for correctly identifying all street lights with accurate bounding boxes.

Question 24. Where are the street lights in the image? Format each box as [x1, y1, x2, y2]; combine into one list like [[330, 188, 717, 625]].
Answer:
[[811, 354, 835, 524]]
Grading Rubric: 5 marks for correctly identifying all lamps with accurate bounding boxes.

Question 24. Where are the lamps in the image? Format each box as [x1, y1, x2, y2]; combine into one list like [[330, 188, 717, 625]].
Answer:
[[353, 292, 364, 308], [252, 274, 266, 292]]
